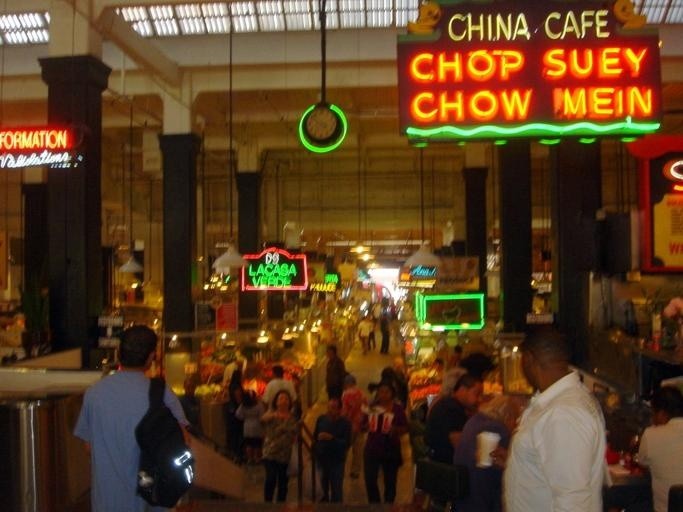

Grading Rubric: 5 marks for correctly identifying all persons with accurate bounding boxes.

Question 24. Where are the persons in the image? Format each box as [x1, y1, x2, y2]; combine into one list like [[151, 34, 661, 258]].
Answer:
[[637, 385, 681, 510], [407, 344, 509, 511], [222, 339, 347, 469], [488, 327, 608, 511], [259, 366, 410, 510], [357, 303, 390, 353], [72, 324, 190, 511]]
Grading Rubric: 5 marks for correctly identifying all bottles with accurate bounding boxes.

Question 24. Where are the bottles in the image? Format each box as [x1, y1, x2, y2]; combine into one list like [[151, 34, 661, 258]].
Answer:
[[631, 435, 640, 465]]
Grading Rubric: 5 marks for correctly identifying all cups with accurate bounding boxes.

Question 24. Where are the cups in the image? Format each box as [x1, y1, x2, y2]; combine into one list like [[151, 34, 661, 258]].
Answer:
[[475, 431, 501, 468]]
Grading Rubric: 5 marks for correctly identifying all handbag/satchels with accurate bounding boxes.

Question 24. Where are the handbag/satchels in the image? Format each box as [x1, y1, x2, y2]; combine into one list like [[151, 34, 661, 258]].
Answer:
[[136, 377, 195, 507]]
